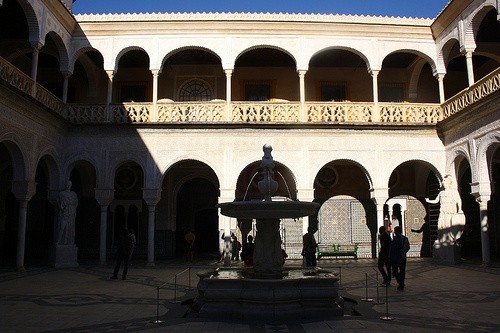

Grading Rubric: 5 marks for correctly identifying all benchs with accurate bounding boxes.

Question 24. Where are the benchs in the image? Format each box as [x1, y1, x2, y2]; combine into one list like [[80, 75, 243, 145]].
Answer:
[[316, 243, 358, 261]]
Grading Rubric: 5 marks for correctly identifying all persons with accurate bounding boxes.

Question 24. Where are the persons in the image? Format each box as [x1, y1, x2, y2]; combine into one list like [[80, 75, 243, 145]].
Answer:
[[241, 235, 256, 269], [231, 235, 242, 262], [301, 226, 320, 268], [111, 222, 136, 281], [391, 226, 410, 290], [184, 228, 197, 262], [377, 225, 392, 287]]
